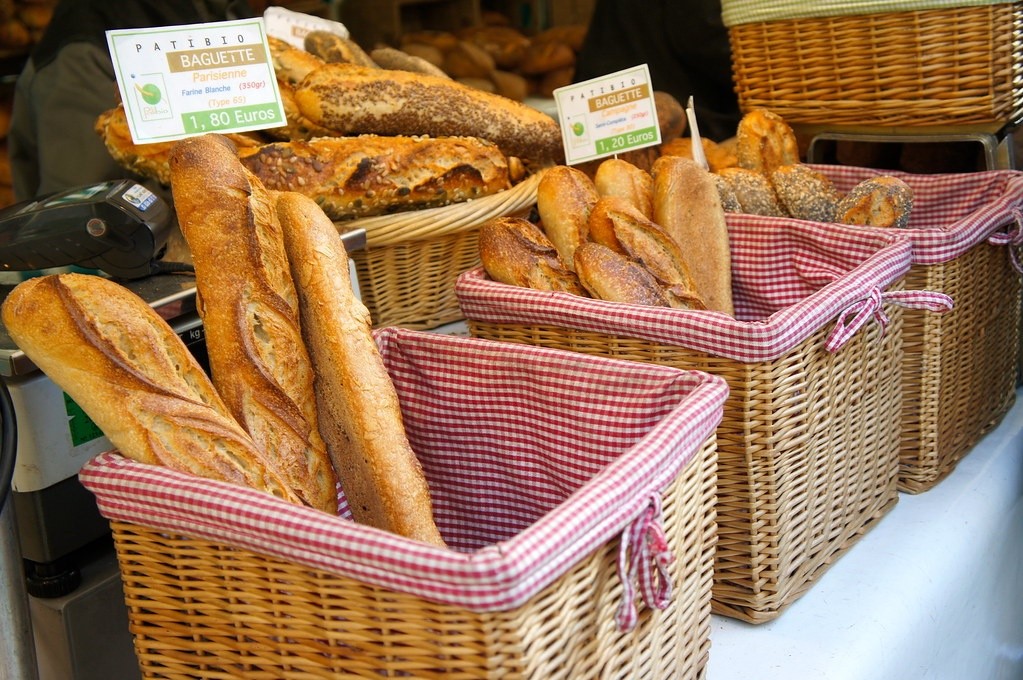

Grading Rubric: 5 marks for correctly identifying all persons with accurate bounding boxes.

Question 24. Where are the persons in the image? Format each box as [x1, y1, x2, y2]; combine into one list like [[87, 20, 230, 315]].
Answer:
[[4, 0, 258, 202]]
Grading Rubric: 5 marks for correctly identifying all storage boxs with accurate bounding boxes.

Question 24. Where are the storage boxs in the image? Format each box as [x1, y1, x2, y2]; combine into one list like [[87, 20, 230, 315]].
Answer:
[[79, 326, 729, 679], [720, 0, 1023, 126], [453, 213, 953, 625], [801, 163, 1023, 494]]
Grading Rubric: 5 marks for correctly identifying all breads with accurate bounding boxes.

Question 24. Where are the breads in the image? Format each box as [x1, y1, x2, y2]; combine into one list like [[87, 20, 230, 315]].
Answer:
[[0, 33, 917, 542]]
